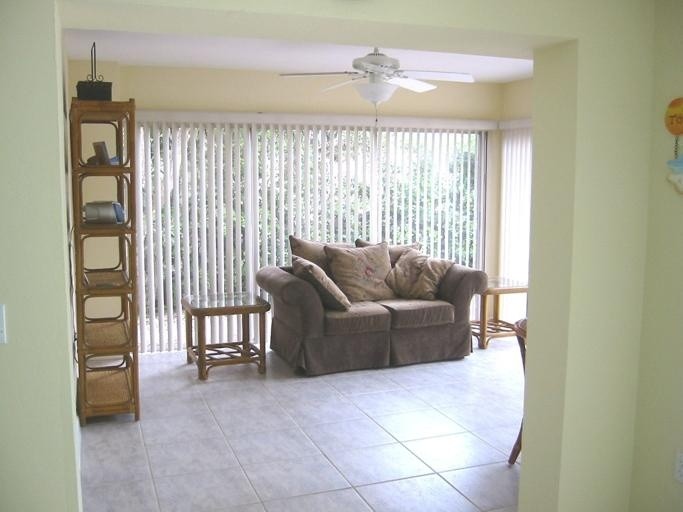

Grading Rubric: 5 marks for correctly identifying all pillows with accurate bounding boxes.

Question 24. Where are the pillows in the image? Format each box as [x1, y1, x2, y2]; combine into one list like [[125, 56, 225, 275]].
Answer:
[[288, 234, 454, 313]]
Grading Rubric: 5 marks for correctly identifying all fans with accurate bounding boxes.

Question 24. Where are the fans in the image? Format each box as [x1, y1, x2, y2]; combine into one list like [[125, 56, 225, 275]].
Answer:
[[277, 48, 474, 124]]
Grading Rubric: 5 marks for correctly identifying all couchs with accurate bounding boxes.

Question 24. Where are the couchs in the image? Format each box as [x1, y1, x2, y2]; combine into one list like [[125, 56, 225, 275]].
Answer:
[[255, 261, 488, 377]]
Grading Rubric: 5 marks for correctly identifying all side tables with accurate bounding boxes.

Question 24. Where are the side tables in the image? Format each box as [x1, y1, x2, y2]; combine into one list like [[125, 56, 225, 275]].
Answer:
[[180, 290, 270, 380], [468, 278, 528, 350]]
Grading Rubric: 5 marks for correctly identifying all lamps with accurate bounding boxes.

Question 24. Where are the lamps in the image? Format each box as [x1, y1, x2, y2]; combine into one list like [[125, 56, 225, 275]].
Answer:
[[352, 82, 398, 110]]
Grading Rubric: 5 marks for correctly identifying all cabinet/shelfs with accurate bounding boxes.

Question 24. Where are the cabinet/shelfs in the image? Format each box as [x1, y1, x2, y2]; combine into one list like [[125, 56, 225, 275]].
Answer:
[[67, 98, 139, 428]]
